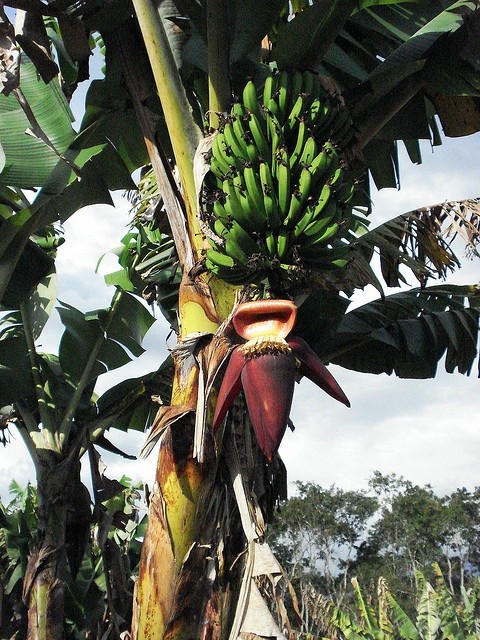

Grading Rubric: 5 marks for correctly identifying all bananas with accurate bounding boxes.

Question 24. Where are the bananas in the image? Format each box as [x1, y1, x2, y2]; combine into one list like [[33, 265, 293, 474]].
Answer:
[[202, 68, 354, 277]]
